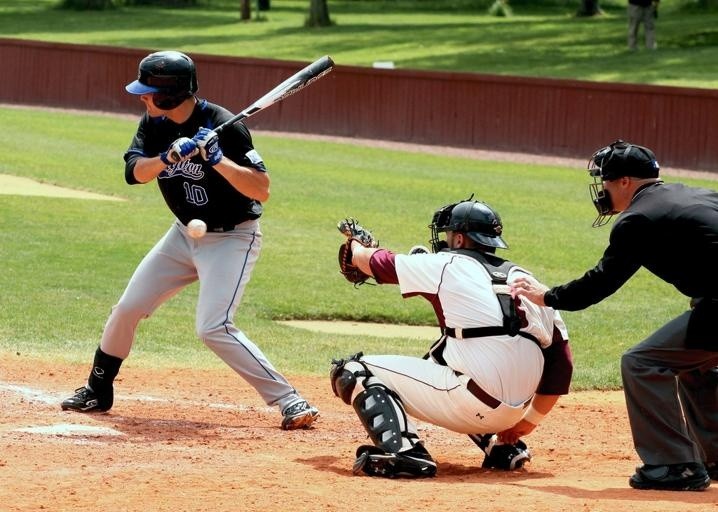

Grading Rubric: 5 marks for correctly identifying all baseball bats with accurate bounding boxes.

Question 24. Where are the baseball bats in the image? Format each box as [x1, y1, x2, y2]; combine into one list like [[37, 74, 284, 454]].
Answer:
[[172, 55, 335, 163]]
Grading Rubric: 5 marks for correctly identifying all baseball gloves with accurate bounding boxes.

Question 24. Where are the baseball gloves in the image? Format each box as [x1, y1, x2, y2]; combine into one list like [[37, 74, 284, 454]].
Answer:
[[336, 219, 379, 286]]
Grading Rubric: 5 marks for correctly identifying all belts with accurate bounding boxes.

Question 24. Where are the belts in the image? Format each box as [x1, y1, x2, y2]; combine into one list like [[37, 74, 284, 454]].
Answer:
[[205, 226, 235, 233], [454, 370, 534, 410]]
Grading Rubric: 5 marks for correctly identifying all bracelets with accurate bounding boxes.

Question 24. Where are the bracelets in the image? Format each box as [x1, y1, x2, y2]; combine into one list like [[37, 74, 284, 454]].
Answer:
[[158, 149, 174, 167]]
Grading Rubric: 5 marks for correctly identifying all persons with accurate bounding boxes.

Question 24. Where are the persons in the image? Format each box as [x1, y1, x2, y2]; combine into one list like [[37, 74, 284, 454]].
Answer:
[[62, 50, 320, 430], [626, 1, 658, 54], [510, 137, 717, 491], [329, 192, 572, 479]]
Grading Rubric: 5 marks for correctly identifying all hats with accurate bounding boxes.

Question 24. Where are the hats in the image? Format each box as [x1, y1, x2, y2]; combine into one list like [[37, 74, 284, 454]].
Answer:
[[586, 140, 661, 228]]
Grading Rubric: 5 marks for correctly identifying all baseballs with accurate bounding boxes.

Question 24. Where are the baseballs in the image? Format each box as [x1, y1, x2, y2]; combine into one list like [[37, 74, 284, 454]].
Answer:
[[188, 220, 207, 237]]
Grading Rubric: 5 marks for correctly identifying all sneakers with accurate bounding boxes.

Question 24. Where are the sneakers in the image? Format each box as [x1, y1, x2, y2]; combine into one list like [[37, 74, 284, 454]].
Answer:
[[354, 440, 438, 478], [701, 462, 718, 483], [279, 400, 321, 430], [60, 388, 116, 412], [627, 462, 709, 492], [480, 435, 530, 468]]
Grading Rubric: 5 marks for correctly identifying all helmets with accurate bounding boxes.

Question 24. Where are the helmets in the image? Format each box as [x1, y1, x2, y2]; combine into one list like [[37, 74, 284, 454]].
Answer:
[[123, 49, 201, 111], [429, 201, 509, 251]]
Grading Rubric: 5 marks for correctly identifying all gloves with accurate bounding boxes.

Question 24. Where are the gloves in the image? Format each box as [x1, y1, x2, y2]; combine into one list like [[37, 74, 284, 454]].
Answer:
[[159, 136, 199, 166], [192, 126, 223, 167]]
